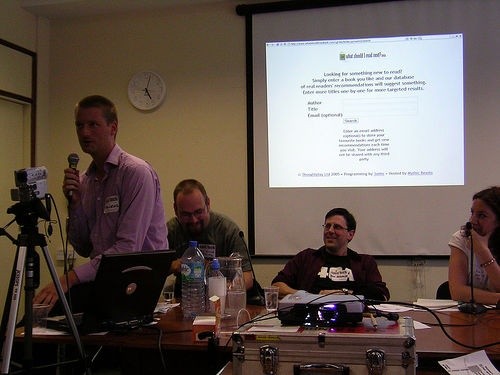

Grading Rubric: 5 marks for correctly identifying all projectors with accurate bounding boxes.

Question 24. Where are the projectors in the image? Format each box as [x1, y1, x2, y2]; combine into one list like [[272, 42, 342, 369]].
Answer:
[[277, 292, 365, 326]]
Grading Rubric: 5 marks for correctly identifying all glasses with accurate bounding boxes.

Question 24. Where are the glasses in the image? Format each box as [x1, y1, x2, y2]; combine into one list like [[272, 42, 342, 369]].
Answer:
[[322, 223, 350, 232], [177, 202, 206, 219]]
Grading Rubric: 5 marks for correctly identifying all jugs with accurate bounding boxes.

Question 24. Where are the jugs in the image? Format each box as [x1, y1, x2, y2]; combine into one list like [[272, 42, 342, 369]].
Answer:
[[204, 253, 246, 317]]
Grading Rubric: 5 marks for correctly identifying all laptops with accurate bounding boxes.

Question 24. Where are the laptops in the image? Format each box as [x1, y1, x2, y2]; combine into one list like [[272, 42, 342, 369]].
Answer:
[[40, 248, 175, 334]]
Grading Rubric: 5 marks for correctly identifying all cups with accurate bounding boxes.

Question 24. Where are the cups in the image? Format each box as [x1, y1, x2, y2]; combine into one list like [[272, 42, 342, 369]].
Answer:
[[32, 304, 50, 333], [163, 280, 174, 302], [264, 286, 280, 311]]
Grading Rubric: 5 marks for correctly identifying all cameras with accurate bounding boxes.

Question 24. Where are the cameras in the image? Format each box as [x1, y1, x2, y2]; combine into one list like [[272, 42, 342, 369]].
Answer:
[[5, 166, 52, 237]]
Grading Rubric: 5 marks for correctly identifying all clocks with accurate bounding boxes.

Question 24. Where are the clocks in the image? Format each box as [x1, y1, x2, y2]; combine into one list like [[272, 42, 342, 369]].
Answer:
[[127, 69, 168, 111]]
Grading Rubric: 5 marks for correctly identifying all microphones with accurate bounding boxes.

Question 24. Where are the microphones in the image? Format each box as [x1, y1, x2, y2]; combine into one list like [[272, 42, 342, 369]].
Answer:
[[239, 231, 266, 305], [67, 153, 79, 208], [457, 222, 487, 315]]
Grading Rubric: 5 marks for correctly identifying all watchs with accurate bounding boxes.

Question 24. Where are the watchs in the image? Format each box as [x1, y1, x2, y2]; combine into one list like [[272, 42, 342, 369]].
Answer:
[[342, 289, 350, 294]]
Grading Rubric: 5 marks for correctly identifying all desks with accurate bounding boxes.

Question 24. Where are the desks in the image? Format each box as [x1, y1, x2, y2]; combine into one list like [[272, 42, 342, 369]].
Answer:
[[12, 304, 500, 375]]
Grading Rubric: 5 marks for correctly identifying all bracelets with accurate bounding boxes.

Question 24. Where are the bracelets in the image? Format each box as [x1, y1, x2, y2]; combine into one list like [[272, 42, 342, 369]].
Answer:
[[481, 256, 495, 268]]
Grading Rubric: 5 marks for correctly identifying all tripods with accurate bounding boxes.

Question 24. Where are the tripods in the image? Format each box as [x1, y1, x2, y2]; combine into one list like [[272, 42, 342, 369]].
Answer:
[[0, 229, 86, 375]]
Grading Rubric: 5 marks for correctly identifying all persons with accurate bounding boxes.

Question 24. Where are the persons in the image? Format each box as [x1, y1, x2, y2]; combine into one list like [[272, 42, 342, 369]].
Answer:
[[165, 178, 254, 299], [448, 186, 500, 308], [269, 207, 391, 305], [10, 95, 171, 330]]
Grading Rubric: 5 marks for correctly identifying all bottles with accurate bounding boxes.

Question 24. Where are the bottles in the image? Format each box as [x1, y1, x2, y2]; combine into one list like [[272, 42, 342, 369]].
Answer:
[[181, 241, 206, 319], [208, 259, 226, 313]]
